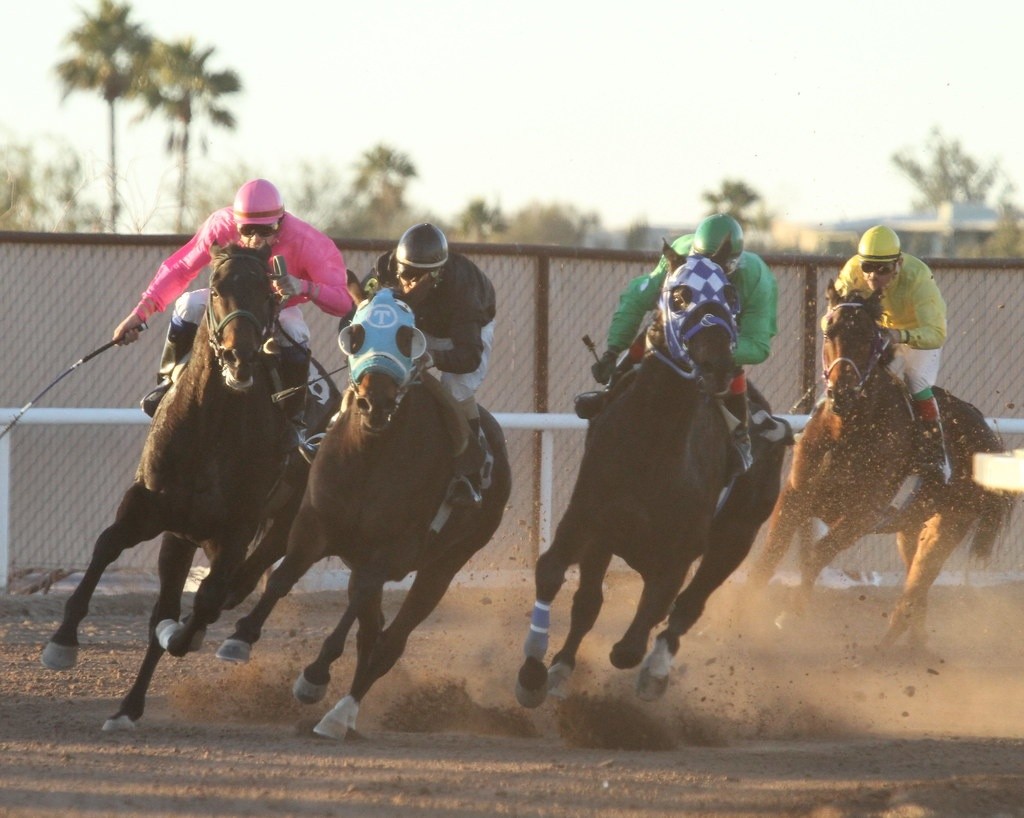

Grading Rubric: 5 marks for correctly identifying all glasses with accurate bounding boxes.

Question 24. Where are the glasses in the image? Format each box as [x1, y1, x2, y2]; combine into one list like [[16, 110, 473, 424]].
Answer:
[[236, 223, 281, 238], [860, 260, 896, 275], [398, 262, 438, 282]]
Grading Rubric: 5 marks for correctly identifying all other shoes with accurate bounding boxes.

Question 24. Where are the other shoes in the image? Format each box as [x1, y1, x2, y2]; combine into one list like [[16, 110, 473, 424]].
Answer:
[[916, 420, 943, 469], [450, 474, 486, 507]]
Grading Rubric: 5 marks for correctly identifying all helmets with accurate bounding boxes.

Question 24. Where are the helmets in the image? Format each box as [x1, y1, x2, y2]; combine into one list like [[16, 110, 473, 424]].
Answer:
[[692, 211, 744, 260], [858, 225, 900, 263], [233, 178, 286, 225], [394, 224, 449, 268]]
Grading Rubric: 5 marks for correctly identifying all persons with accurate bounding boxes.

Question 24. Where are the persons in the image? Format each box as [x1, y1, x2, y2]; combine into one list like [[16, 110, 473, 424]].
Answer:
[[297, 214, 496, 510], [592, 214, 777, 475], [113, 177, 354, 461], [820, 225, 946, 443]]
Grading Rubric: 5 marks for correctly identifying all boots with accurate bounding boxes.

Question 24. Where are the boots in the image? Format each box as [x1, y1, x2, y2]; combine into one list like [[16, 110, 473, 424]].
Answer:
[[276, 345, 311, 457], [722, 391, 751, 478], [141, 308, 200, 415]]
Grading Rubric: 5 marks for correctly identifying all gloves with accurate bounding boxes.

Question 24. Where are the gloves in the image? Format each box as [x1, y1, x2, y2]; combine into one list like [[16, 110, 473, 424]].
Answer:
[[590, 350, 615, 386]]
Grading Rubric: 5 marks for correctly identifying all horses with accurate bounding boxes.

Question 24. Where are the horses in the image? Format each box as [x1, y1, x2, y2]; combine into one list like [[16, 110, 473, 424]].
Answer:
[[39, 242, 330, 732], [217, 268, 513, 743], [735, 278, 1015, 664], [515, 237, 788, 710]]
